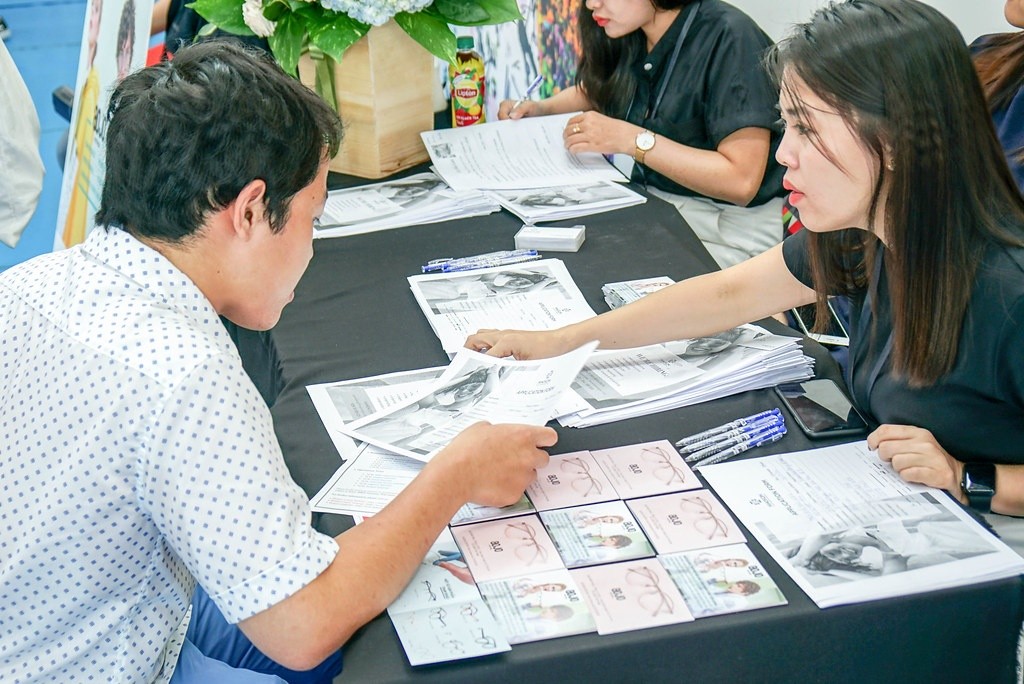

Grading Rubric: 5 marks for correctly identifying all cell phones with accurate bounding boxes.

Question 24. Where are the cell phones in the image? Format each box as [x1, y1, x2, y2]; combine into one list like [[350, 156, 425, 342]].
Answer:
[[792, 299, 850, 346], [776, 382, 869, 439]]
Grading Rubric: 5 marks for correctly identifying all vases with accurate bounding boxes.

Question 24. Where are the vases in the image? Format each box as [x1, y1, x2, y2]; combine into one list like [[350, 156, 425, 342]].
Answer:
[[291, 11, 433, 182]]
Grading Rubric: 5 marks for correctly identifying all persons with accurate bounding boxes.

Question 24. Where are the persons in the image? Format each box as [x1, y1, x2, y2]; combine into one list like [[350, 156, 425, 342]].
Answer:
[[0, 42, 559, 683], [462, 1, 1023, 517], [498, 1, 793, 271], [149, 1, 275, 56]]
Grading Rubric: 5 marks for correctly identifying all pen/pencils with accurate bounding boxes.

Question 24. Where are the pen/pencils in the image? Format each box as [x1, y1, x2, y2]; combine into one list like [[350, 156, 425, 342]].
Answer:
[[679, 412, 782, 454], [421, 249, 542, 273], [685, 421, 784, 463], [673, 407, 780, 447], [510, 73, 544, 112], [691, 427, 786, 471]]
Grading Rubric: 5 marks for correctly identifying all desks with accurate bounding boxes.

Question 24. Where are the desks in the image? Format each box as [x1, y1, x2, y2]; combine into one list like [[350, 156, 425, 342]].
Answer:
[[215, 143, 1024, 684]]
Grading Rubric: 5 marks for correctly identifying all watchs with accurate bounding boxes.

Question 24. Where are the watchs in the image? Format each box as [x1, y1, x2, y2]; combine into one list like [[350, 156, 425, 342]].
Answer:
[[960, 460, 996, 514], [634, 129, 656, 164]]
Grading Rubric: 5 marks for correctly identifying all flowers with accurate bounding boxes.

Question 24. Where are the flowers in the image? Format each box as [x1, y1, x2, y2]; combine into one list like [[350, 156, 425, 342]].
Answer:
[[184, 0, 526, 161]]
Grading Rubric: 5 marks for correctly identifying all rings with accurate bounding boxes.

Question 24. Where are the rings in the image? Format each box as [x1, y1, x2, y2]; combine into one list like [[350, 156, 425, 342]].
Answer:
[[572, 123, 581, 134]]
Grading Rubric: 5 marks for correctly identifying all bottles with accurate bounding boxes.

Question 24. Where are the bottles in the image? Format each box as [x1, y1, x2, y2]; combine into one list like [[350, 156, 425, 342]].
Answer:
[[448, 36, 485, 127]]
